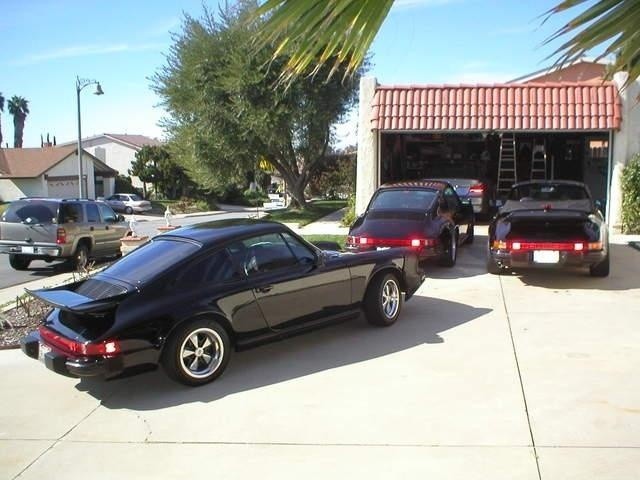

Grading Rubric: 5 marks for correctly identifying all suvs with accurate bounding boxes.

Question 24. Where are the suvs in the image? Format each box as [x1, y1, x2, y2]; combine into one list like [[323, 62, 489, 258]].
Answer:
[[0, 196, 131, 271]]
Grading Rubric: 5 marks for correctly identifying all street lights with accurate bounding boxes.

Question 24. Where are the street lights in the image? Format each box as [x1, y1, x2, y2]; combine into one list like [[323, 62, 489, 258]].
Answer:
[[75, 75, 104, 199]]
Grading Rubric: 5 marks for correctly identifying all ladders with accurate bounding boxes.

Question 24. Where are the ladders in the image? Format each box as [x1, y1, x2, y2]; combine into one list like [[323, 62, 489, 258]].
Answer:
[[496, 132, 517, 192], [530, 134, 548, 180]]
